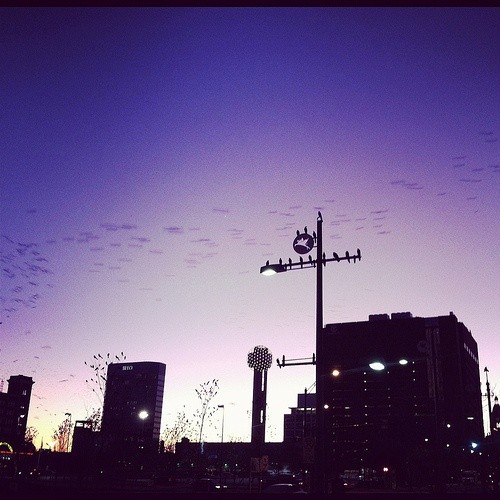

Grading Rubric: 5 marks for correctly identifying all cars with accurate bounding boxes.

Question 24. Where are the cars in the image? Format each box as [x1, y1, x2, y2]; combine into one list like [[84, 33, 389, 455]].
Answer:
[[7, 457, 394, 487]]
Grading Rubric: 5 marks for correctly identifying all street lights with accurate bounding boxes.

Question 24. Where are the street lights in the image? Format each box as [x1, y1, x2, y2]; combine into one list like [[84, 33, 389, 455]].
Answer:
[[249, 211, 364, 424], [137, 410, 148, 474]]
